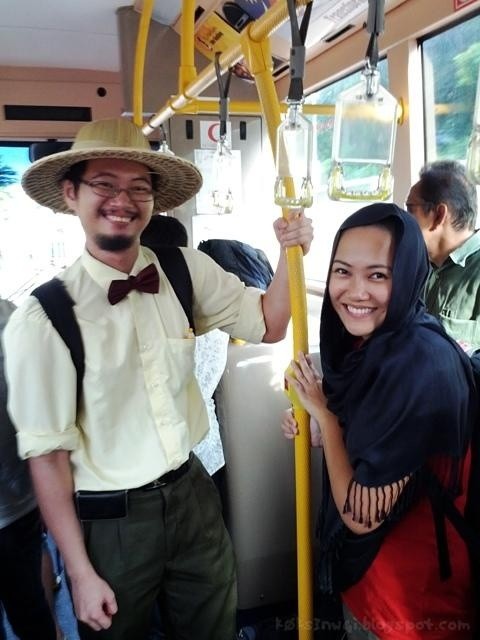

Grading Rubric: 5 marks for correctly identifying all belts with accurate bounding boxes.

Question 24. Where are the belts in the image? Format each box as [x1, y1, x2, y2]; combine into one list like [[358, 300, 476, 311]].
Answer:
[[130, 451, 195, 492]]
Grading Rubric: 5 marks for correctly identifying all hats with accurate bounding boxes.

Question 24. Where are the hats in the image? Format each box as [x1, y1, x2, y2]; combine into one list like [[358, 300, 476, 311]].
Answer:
[[21, 116, 203, 217]]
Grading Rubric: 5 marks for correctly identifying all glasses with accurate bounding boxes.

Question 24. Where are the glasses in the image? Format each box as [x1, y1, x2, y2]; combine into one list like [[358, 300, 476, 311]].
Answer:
[[73, 174, 158, 202]]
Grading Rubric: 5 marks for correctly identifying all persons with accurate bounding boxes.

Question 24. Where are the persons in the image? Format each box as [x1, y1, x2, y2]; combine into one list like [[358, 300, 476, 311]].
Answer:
[[1, 297, 66, 638], [140, 212, 229, 551], [406, 158, 478, 365], [283, 203, 477, 639], [0, 155, 317, 640]]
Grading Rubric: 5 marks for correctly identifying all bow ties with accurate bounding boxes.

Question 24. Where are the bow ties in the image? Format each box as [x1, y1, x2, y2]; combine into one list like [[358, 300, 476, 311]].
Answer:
[[107, 263, 161, 306]]
